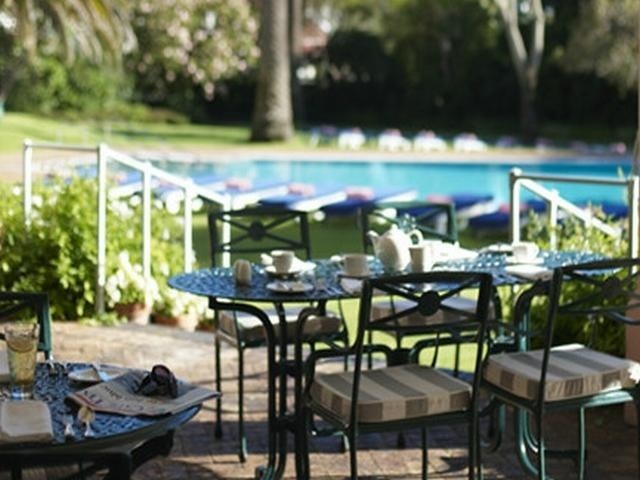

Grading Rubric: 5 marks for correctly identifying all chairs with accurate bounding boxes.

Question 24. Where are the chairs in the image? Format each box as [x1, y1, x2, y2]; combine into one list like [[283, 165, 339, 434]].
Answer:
[[482, 260, 639, 478], [208, 209, 348, 463], [1, 290, 51, 379], [302, 272, 493, 480], [357, 201, 497, 446]]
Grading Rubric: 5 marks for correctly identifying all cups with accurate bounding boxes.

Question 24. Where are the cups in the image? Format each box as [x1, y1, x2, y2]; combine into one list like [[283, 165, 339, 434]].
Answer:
[[271, 249, 295, 272], [4, 321, 40, 397], [337, 254, 367, 273], [511, 241, 539, 262], [235, 260, 252, 287], [409, 247, 423, 271]]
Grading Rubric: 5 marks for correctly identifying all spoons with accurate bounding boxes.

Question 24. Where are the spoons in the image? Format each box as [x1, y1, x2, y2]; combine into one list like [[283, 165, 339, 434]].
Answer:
[[76, 405, 96, 437]]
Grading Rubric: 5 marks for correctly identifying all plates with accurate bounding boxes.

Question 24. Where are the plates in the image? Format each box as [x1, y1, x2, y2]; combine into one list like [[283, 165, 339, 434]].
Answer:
[[264, 266, 303, 278], [504, 257, 545, 265], [265, 281, 314, 294], [336, 271, 371, 277], [504, 266, 548, 279], [68, 367, 124, 383]]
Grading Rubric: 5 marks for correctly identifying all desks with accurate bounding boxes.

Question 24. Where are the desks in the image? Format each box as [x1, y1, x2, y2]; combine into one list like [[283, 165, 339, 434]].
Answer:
[[0, 368, 202, 480], [167, 249, 609, 479]]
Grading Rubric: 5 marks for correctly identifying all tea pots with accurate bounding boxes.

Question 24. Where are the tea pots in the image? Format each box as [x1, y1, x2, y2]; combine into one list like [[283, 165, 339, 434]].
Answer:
[[365, 223, 424, 271]]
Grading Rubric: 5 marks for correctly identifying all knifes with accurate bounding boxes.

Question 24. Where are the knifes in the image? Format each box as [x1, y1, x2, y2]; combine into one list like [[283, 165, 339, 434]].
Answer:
[[91, 361, 109, 383]]
